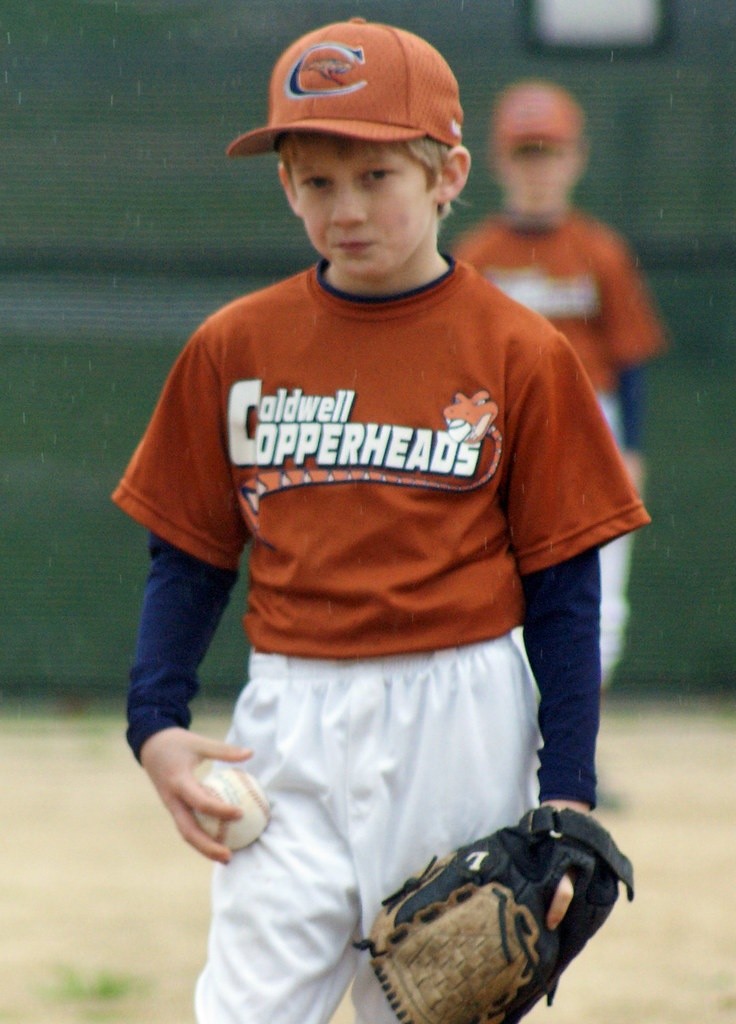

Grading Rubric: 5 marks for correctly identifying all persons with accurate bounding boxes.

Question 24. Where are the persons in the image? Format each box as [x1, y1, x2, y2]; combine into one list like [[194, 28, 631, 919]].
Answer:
[[112, 13, 649, 1024], [451, 81, 664, 747]]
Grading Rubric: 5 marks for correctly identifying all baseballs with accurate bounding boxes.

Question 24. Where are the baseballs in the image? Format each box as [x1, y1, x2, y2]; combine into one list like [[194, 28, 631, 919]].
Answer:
[[194, 770, 274, 851]]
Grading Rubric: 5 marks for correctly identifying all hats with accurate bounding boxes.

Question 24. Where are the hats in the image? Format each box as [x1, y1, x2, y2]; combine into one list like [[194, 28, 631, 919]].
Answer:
[[491, 83, 580, 152], [227, 16, 463, 158]]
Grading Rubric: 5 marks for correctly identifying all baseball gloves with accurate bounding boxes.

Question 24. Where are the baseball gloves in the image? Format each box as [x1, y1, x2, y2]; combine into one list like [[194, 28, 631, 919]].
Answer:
[[351, 805, 634, 1024]]
